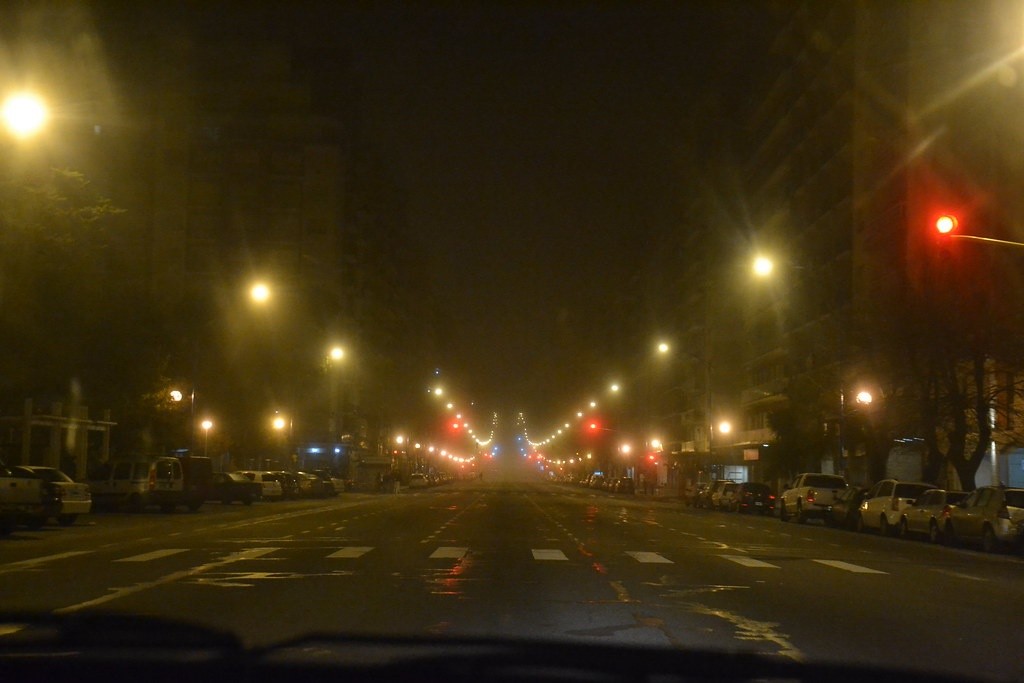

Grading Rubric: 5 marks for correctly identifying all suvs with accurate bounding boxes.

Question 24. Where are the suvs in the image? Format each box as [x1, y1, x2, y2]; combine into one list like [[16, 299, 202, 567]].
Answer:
[[943, 484, 1023, 551], [779, 472, 848, 523], [853, 477, 938, 536]]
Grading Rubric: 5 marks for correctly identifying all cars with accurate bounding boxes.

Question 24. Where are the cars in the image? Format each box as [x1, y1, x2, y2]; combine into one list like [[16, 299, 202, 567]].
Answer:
[[0, 464, 91, 523], [537, 467, 777, 515], [828, 486, 869, 526], [900, 489, 972, 542], [124, 451, 476, 509]]
